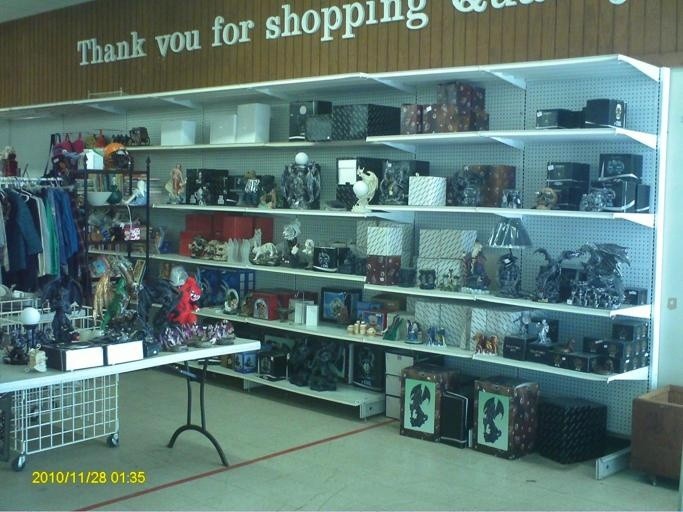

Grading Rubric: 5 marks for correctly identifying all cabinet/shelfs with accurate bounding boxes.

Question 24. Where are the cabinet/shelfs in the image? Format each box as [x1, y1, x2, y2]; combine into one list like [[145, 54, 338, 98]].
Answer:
[[84, 71, 415, 422], [362, 53, 671, 481]]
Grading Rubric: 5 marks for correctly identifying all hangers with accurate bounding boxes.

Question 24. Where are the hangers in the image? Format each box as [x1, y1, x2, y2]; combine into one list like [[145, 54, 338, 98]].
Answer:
[[0, 176, 32, 203]]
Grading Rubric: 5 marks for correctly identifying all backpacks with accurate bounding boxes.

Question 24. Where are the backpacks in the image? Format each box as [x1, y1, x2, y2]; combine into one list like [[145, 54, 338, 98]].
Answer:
[[53, 129, 107, 155]]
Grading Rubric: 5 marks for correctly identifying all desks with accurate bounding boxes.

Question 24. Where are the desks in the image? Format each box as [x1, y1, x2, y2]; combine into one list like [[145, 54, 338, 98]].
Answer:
[[0, 338, 261, 467]]
